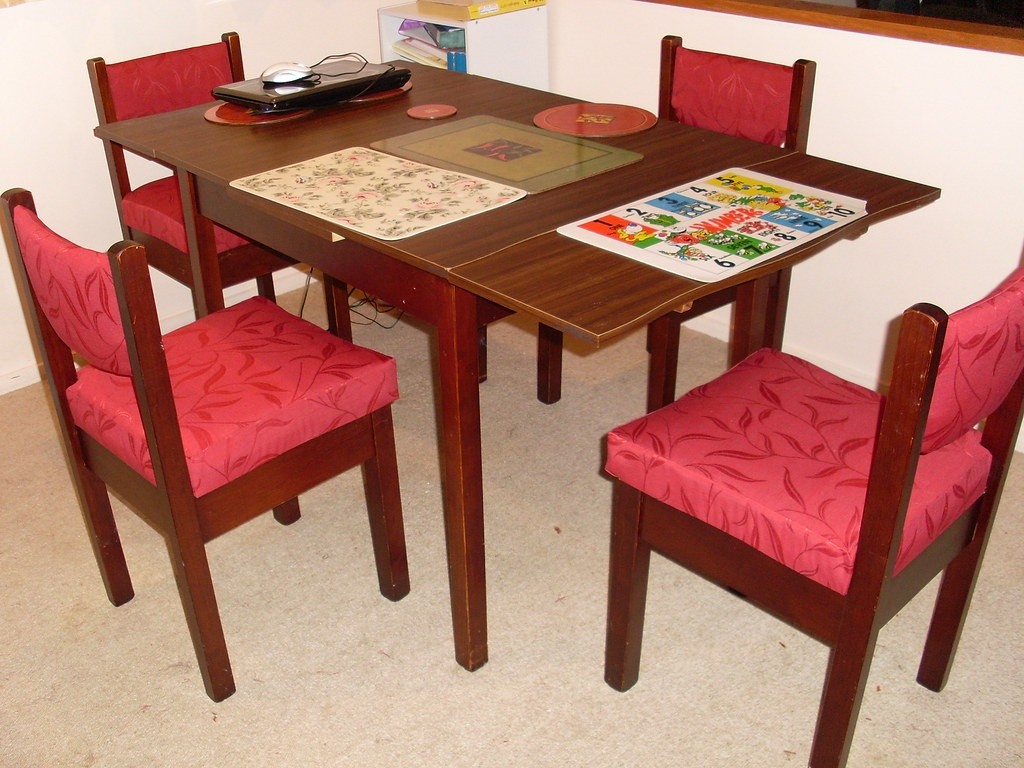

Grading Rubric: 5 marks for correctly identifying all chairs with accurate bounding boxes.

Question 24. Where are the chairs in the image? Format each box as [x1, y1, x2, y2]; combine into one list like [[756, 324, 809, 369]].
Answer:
[[85, 29, 355, 341], [0, 181, 411, 701], [537, 36, 816, 414], [594, 246, 1024, 768]]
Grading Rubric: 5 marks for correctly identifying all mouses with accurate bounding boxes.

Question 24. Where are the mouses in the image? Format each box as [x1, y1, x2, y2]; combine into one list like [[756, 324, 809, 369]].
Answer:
[[262, 61, 315, 84]]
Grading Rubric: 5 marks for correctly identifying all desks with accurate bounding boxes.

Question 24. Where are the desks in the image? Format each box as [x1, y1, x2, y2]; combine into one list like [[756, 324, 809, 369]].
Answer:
[[93, 59, 943, 673]]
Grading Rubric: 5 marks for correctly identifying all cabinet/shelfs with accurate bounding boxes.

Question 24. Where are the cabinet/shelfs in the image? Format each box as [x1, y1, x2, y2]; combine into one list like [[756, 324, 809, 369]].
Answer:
[[381, 3, 549, 91]]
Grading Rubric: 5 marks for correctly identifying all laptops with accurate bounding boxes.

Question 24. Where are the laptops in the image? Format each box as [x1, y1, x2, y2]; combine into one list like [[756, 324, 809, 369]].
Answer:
[[211, 59, 412, 113]]
